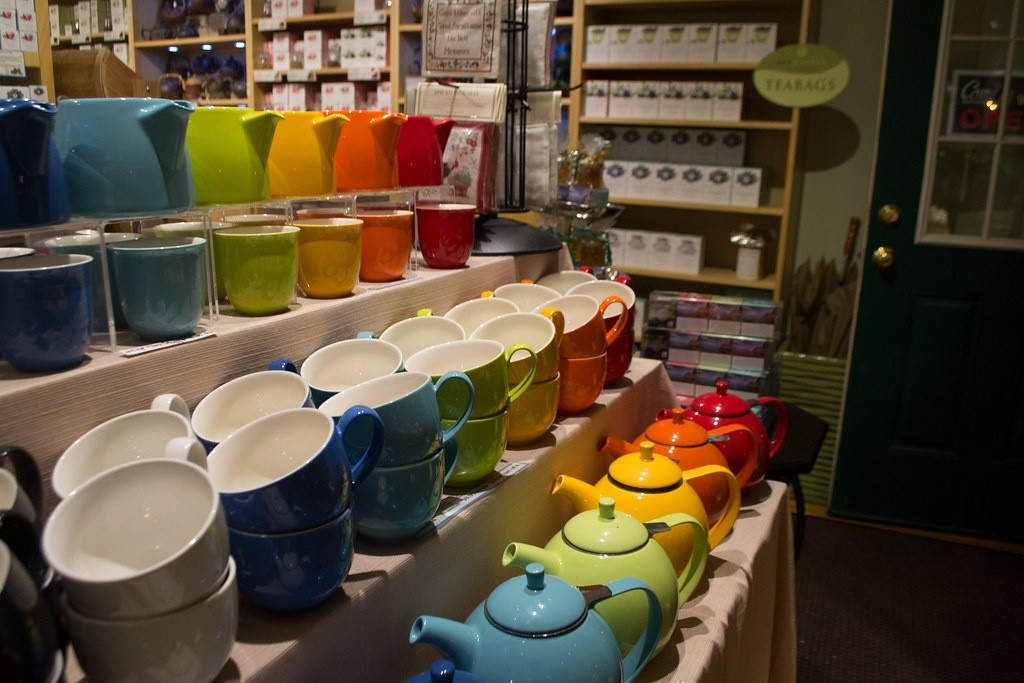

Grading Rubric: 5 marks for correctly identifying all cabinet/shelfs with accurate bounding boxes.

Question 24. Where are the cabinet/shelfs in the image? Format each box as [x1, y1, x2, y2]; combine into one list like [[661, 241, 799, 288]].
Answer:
[[0, 0, 799, 683]]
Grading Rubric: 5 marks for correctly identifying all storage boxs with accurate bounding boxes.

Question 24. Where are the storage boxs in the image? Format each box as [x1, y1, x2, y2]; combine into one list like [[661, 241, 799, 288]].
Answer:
[[718, 24, 744, 63], [583, 78, 609, 119], [660, 24, 688, 64], [679, 165, 704, 203], [609, 80, 631, 119], [745, 22, 776, 63], [688, 23, 719, 63], [703, 164, 733, 206], [711, 83, 744, 121], [731, 166, 763, 207], [658, 79, 686, 120], [627, 160, 652, 200], [610, 26, 636, 63], [635, 23, 659, 65], [633, 82, 659, 120], [586, 24, 611, 64], [651, 162, 680, 202], [685, 82, 712, 119]]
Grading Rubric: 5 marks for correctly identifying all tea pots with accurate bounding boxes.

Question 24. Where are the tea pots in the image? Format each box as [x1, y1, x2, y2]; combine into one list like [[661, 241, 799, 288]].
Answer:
[[0, 19, 458, 229], [402, 380, 788, 682]]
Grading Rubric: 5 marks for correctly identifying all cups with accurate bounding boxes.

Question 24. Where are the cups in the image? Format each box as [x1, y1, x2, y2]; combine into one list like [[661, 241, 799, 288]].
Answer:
[[0, 208, 636, 683]]
